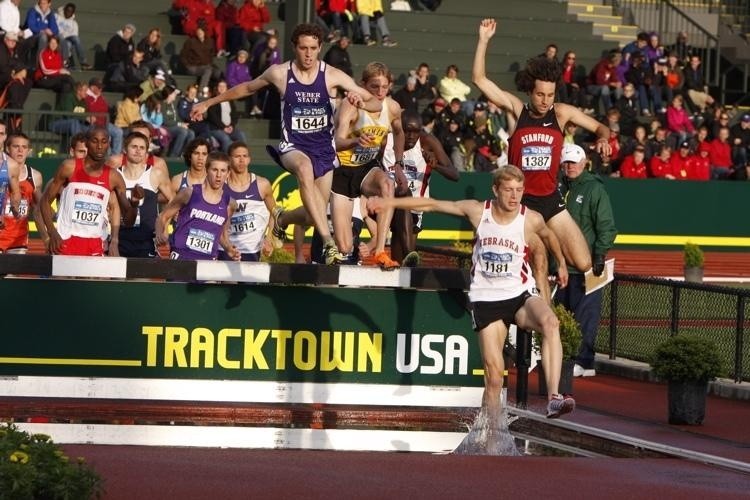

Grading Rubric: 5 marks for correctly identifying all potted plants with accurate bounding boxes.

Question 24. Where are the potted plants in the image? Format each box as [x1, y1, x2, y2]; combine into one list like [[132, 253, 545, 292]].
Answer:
[[680, 238, 709, 285], [533, 304, 584, 398], [648, 332, 726, 429]]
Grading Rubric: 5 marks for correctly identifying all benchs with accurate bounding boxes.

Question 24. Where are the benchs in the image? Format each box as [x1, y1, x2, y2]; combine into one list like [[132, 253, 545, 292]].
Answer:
[[1, 1, 638, 165], [642, 1, 747, 87]]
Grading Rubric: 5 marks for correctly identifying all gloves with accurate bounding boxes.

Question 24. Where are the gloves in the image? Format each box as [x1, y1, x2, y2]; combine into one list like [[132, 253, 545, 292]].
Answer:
[[592, 253, 605, 277]]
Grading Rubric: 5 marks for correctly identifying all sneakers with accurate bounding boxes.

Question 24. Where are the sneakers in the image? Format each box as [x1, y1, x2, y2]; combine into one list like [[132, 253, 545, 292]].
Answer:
[[363, 38, 397, 48], [572, 364, 584, 376], [582, 362, 597, 378], [270, 204, 288, 251], [545, 391, 575, 420], [319, 240, 419, 270]]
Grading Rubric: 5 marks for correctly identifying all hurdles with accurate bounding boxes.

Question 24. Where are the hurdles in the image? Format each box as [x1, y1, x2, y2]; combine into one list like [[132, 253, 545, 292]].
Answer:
[[0, 253, 532, 409]]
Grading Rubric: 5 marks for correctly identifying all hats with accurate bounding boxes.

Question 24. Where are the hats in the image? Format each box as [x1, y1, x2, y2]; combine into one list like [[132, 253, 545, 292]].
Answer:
[[680, 141, 691, 149], [737, 112, 749, 122], [560, 142, 586, 166]]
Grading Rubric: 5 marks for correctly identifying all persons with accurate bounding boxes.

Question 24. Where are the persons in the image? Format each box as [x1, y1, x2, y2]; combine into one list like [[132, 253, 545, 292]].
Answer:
[[551, 143, 618, 377], [470, 16, 613, 307], [366, 164, 575, 455]]
